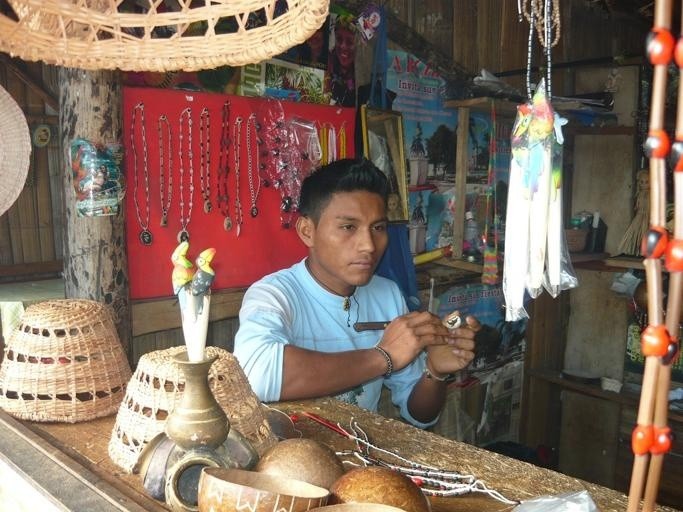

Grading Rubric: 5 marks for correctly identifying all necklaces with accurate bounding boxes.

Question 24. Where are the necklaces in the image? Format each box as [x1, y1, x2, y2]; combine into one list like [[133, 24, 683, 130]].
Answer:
[[130, 98, 348, 248], [305, 260, 360, 310]]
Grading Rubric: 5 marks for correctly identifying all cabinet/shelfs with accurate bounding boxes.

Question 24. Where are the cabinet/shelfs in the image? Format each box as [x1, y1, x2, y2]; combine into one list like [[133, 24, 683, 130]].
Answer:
[[413, 95, 683, 508]]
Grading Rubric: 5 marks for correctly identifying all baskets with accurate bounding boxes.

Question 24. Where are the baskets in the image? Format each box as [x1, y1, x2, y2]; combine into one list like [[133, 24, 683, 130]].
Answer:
[[0, 86, 32, 219], [565, 229, 588, 254], [0, 0, 329, 72], [108, 345, 278, 473], [0, 300, 133, 423]]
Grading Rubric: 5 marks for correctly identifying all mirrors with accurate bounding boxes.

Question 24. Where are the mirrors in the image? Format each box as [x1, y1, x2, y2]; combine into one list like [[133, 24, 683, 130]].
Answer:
[[360, 105, 409, 224]]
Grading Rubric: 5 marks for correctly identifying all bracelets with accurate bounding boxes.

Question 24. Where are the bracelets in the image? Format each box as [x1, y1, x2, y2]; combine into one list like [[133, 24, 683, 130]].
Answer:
[[423, 361, 451, 385], [373, 345, 392, 378]]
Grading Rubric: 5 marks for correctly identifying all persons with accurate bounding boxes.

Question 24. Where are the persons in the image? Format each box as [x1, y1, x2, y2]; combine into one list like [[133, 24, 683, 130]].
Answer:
[[230, 157, 483, 430]]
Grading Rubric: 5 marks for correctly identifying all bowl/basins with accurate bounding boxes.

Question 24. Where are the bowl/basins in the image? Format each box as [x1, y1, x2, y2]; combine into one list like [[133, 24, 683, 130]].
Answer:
[[196, 467, 330, 512]]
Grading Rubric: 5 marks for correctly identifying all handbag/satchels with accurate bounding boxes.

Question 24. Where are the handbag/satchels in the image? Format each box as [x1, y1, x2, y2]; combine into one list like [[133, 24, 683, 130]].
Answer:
[[481, 247, 498, 285]]
